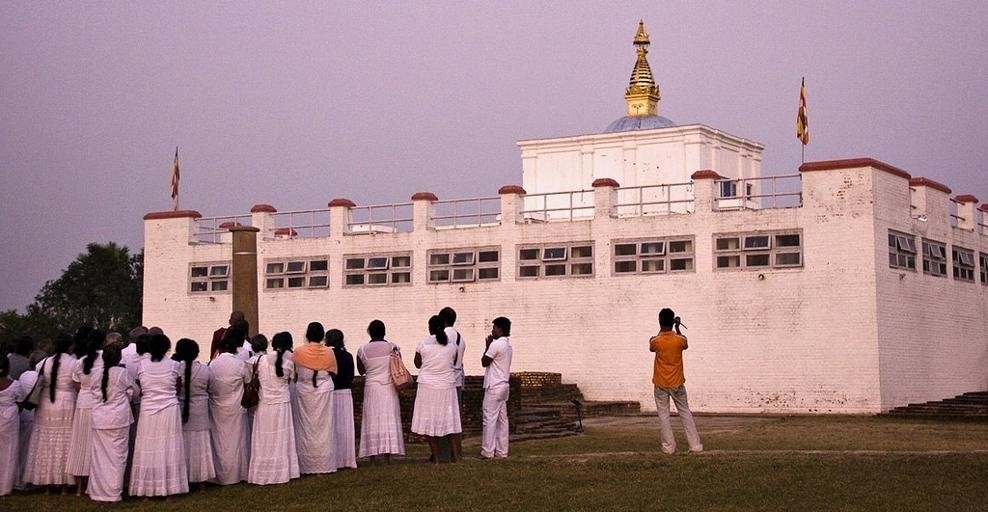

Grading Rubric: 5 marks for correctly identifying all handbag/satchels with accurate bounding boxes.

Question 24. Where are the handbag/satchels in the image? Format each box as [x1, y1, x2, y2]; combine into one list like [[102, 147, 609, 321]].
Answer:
[[390, 349, 413, 389], [240, 377, 259, 407], [24, 372, 45, 411]]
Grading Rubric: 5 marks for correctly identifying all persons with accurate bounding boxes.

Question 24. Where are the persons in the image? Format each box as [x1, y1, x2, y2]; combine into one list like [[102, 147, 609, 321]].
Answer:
[[477, 317, 512, 459], [0, 311, 357, 502], [410, 315, 463, 465], [650, 308, 704, 456], [441, 306, 466, 463], [356, 319, 407, 465]]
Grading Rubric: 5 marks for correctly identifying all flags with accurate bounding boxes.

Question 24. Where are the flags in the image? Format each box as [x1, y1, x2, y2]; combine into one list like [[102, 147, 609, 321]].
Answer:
[[797, 78, 809, 148], [170, 149, 180, 212]]
